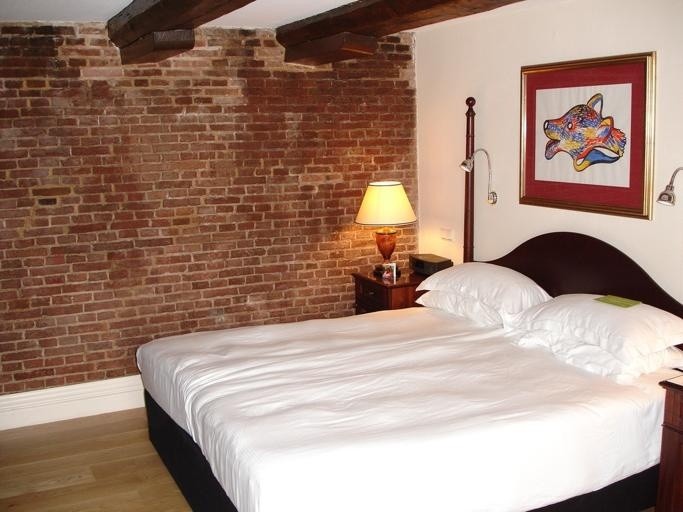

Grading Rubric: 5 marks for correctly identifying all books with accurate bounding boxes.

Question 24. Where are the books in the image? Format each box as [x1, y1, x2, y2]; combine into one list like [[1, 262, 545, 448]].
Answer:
[[594, 294, 641, 310]]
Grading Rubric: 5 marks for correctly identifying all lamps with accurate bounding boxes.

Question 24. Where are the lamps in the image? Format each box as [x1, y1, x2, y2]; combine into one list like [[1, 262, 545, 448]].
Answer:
[[354, 180, 417, 279], [459, 148, 497, 204], [657, 167, 682, 206]]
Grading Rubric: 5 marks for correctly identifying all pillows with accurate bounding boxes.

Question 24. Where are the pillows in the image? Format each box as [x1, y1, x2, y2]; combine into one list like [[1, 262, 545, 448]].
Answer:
[[415, 261, 683, 388]]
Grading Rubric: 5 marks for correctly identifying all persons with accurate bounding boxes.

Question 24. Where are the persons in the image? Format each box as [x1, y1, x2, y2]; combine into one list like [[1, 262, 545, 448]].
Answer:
[[382, 266, 393, 279]]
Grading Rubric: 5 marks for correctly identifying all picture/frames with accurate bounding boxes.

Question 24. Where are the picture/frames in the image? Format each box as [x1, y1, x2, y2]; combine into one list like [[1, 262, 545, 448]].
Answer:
[[381, 262, 396, 285], [519, 52, 656, 221]]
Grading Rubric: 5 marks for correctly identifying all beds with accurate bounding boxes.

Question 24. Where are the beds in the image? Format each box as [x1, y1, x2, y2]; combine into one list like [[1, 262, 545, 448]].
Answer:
[[137, 231, 683, 512]]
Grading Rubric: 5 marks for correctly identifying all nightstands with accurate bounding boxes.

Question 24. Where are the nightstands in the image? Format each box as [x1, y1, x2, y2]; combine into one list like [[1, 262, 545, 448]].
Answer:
[[350, 267, 430, 315], [655, 374, 683, 512]]
